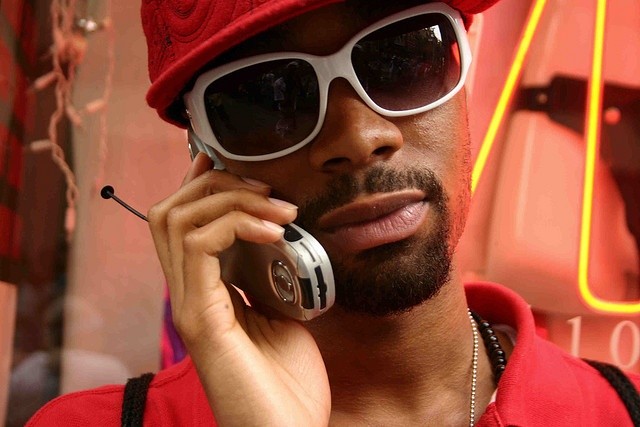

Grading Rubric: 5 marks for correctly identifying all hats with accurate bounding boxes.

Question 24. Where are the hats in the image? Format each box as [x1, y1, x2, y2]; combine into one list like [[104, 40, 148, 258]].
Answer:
[[140, 0, 500, 129]]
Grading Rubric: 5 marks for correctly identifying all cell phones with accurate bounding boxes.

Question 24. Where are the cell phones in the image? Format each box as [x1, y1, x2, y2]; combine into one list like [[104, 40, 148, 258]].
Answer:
[[101, 114, 336, 322]]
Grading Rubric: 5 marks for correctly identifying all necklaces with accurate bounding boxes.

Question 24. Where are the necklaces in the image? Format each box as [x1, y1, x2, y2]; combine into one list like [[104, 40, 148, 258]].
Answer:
[[472, 311, 507, 385], [466, 303, 479, 425]]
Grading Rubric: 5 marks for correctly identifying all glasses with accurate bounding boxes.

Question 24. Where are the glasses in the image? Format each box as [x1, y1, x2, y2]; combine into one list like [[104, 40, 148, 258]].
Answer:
[[183, 1, 471, 161]]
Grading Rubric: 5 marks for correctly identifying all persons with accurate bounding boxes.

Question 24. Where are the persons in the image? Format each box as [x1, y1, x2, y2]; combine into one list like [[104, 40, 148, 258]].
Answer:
[[24, 0, 639, 426]]
